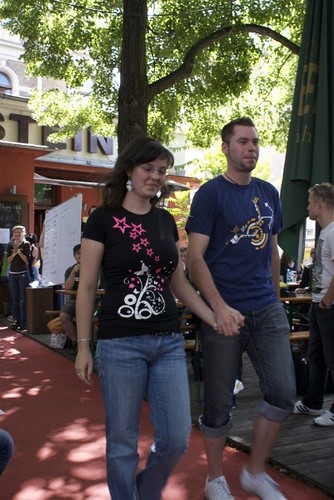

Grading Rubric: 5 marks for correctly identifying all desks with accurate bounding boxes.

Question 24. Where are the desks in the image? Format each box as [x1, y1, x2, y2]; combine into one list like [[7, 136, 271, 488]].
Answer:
[[53, 280, 316, 323]]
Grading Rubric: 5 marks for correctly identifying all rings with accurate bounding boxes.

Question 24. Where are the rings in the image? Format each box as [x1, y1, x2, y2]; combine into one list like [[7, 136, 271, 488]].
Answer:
[[77, 373, 80, 376]]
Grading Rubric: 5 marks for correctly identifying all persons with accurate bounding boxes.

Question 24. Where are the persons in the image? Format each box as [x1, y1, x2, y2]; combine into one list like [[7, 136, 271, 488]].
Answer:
[[278, 251, 297, 282], [304, 249, 315, 268], [59, 244, 82, 353], [25, 233, 39, 280], [6, 226, 31, 330], [73, 138, 240, 500], [179, 247, 191, 281], [292, 183, 334, 425], [183, 119, 296, 500]]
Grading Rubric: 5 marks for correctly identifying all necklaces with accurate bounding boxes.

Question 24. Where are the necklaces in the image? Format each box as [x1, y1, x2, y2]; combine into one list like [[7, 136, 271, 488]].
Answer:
[[225, 173, 240, 186]]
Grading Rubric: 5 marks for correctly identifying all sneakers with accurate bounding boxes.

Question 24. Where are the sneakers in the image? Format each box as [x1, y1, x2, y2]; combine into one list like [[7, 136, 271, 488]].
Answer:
[[239, 465, 287, 500], [312, 411, 334, 426], [292, 400, 323, 416], [203, 475, 234, 500]]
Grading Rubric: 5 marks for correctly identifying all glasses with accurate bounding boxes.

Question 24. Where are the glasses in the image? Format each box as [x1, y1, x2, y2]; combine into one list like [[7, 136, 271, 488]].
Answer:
[[312, 182, 322, 194], [136, 162, 167, 176]]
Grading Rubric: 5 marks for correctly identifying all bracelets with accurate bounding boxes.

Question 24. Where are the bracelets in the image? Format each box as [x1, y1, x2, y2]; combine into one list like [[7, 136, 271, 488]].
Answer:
[[77, 338, 91, 343]]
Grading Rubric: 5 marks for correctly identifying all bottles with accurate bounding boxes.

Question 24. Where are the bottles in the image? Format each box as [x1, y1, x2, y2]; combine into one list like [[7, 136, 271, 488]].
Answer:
[[286, 269, 292, 284]]
